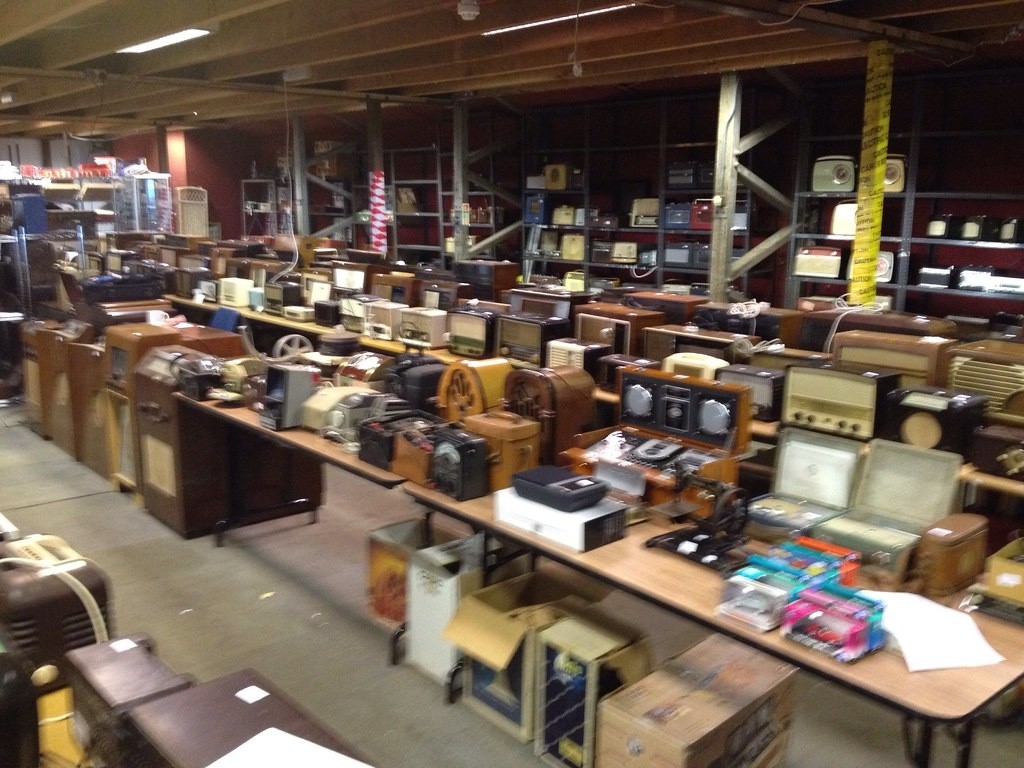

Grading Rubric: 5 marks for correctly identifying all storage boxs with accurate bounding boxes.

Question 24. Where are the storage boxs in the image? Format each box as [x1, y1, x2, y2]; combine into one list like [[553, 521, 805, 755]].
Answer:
[[366, 519, 471, 624], [493, 487, 631, 552], [405, 532, 503, 686], [533, 607, 656, 768], [594, 632, 800, 768], [988, 537, 1024, 603], [442, 562, 618, 745]]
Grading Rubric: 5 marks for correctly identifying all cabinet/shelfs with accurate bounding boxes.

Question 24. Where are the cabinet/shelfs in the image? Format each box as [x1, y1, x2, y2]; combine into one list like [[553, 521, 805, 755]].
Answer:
[[519, 88, 781, 303], [436, 112, 519, 273], [350, 147, 441, 267], [240, 178, 349, 239], [784, 67, 1024, 327]]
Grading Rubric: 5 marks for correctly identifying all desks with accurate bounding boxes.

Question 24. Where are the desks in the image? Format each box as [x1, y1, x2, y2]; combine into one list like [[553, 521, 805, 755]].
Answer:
[[385, 473, 1024, 768], [174, 387, 412, 665], [163, 292, 367, 345], [359, 336, 474, 365], [593, 388, 1024, 499]]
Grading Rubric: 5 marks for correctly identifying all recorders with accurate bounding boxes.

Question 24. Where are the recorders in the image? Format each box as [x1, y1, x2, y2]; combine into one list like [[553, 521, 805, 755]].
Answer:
[[103, 154, 1024, 502]]
